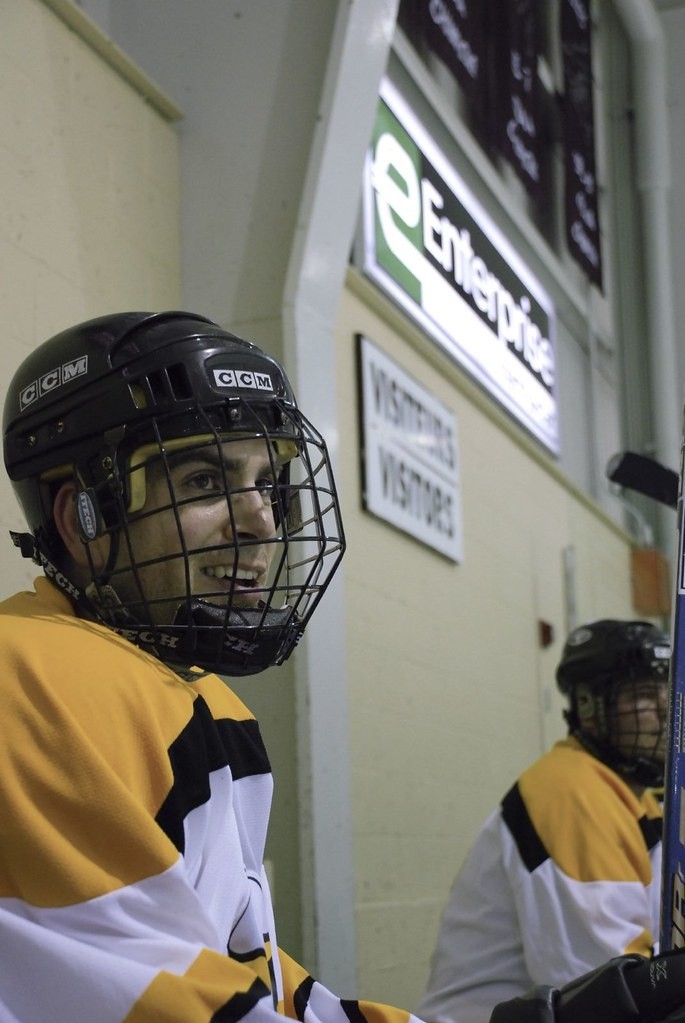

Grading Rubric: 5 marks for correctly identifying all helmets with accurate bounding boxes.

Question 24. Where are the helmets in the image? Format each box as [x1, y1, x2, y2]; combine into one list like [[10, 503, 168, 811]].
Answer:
[[555, 618, 672, 788], [3, 310, 346, 678]]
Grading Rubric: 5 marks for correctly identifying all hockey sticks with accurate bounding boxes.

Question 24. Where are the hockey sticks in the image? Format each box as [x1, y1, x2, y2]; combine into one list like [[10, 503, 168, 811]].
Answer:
[[606, 448, 684, 953]]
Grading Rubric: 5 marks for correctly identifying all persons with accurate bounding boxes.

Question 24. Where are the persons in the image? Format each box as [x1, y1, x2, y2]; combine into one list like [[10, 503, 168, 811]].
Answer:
[[0, 310, 426, 1023], [418, 619, 685, 1023]]
